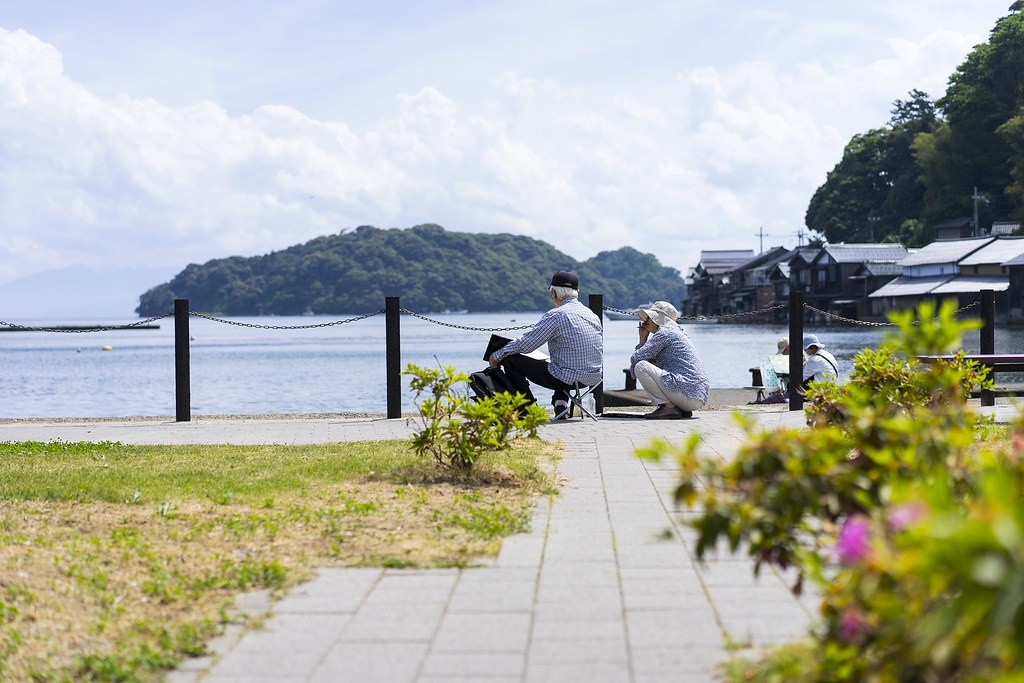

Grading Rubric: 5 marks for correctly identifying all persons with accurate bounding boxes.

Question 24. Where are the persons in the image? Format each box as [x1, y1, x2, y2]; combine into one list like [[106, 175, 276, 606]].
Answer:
[[488, 269, 604, 420], [629, 300, 710, 419], [775, 334, 838, 402]]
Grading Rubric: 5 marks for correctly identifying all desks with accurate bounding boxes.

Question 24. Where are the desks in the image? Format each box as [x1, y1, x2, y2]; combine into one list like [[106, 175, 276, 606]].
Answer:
[[916, 354, 1024, 406]]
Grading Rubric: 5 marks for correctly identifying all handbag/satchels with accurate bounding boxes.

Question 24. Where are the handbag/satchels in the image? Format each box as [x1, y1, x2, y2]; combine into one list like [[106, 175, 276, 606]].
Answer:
[[468, 366, 511, 425]]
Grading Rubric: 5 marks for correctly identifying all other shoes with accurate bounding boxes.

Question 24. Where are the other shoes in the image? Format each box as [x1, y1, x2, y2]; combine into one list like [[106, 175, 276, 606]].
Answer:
[[554, 400, 568, 419]]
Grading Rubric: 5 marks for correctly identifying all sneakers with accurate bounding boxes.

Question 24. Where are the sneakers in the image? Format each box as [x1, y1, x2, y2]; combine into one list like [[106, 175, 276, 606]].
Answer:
[[645, 404, 692, 419]]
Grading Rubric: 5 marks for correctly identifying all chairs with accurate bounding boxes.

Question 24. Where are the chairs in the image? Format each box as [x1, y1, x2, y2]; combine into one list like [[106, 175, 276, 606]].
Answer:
[[552, 370, 603, 422]]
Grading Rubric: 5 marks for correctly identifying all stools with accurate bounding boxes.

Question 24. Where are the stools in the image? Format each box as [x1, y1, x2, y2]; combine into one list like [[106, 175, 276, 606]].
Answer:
[[743, 386, 766, 404]]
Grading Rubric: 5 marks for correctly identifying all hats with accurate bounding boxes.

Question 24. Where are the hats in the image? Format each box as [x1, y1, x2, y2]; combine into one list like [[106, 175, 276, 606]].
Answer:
[[803, 334, 825, 350], [549, 270, 579, 290], [776, 337, 789, 355], [639, 301, 678, 326]]
